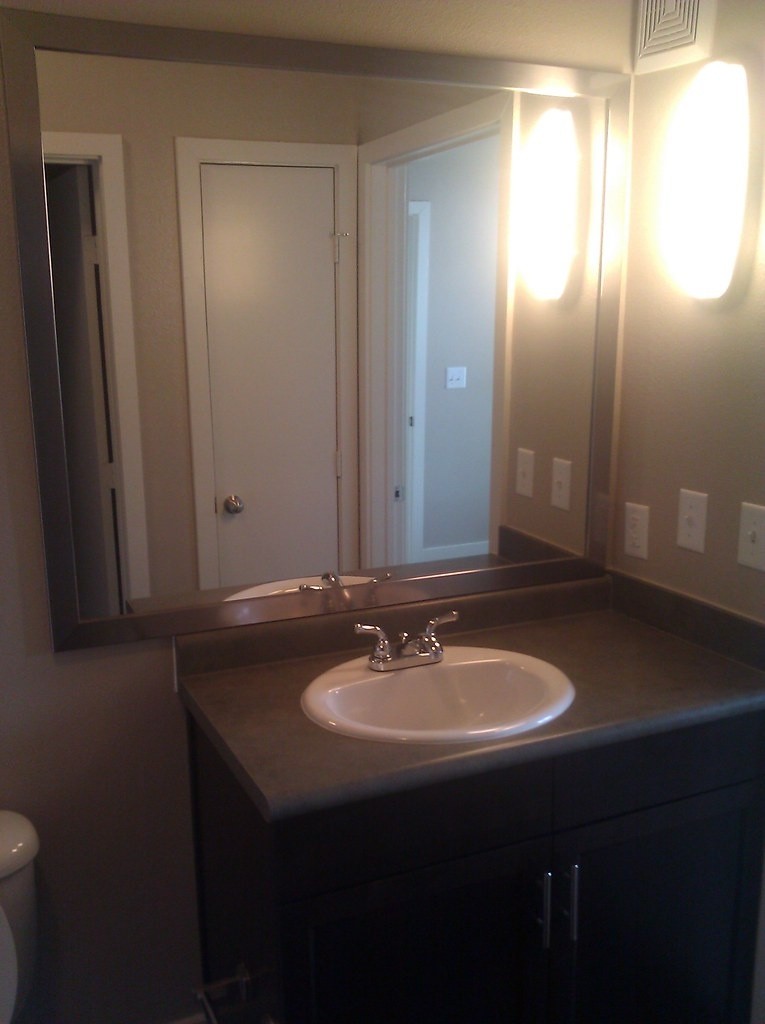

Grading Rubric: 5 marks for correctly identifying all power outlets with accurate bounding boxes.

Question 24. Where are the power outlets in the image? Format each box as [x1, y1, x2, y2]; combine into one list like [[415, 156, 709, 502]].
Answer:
[[624, 502, 649, 560], [446, 367, 466, 389]]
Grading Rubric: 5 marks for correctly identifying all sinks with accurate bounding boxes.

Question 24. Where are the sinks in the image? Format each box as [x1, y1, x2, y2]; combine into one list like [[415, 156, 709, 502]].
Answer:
[[223, 575, 376, 601], [299, 644, 578, 745]]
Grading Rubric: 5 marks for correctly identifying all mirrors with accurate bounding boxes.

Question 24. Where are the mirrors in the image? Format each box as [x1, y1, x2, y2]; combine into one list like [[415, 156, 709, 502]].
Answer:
[[0, 8, 635, 655]]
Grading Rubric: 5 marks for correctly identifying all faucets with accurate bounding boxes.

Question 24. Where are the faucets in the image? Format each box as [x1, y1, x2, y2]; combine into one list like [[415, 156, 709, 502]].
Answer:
[[321, 572, 344, 588], [397, 631, 444, 669]]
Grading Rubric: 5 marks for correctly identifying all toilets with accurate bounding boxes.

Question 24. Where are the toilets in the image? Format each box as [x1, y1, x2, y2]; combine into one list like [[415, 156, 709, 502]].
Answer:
[[1, 809, 41, 1024]]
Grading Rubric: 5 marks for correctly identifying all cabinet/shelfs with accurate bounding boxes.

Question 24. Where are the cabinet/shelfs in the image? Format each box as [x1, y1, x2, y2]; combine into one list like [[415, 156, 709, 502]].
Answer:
[[184, 709, 765, 1024]]
